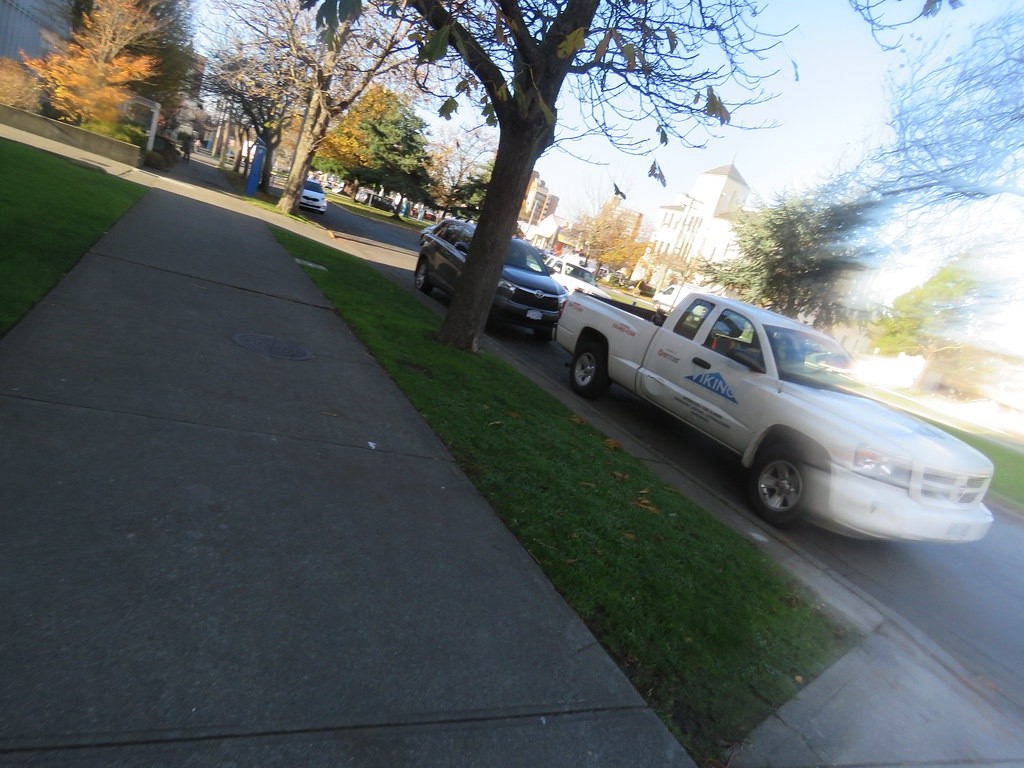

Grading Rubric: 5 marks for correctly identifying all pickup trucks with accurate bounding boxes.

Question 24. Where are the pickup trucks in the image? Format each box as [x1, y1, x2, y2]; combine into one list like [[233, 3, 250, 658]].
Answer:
[[555, 288, 995, 543]]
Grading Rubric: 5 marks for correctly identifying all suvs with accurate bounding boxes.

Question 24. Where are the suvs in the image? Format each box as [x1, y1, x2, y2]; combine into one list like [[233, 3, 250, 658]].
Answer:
[[414, 215, 571, 342]]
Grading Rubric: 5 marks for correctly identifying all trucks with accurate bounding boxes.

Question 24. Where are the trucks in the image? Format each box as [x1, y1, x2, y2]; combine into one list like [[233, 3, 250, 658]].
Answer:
[[651, 284, 709, 312]]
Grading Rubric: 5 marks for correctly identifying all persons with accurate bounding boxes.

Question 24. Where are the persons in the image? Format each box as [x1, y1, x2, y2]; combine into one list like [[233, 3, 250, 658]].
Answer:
[[182, 135, 203, 159]]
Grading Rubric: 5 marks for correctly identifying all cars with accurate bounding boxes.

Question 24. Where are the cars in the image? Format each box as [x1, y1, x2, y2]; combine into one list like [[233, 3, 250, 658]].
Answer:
[[320, 180, 440, 223], [299, 179, 327, 214], [530, 256, 612, 300]]
